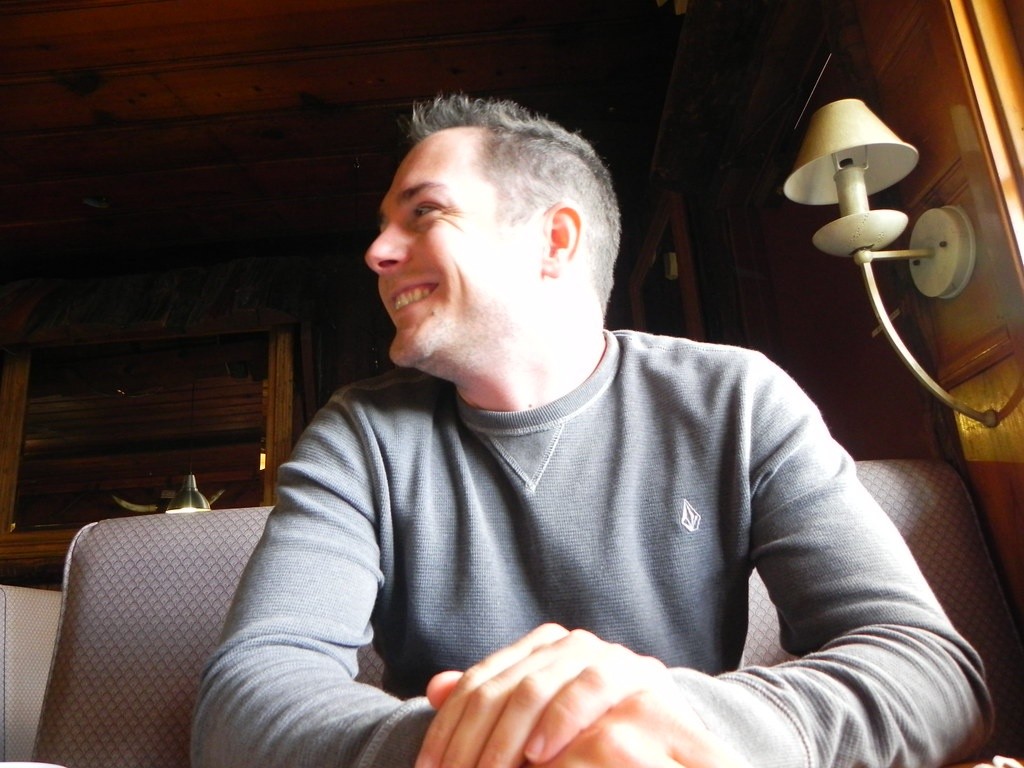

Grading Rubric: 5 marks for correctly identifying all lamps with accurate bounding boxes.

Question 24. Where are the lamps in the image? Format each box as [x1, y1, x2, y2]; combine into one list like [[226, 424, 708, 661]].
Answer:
[[164, 343, 211, 513], [783, 97, 1000, 430]]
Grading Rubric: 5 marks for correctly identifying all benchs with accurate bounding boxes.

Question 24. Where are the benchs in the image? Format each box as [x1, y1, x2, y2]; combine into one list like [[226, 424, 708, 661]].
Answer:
[[0, 456, 1024, 768]]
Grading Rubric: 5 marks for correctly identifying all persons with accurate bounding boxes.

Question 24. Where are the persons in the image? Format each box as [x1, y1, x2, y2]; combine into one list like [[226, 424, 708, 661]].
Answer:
[[190, 93, 995, 768]]
